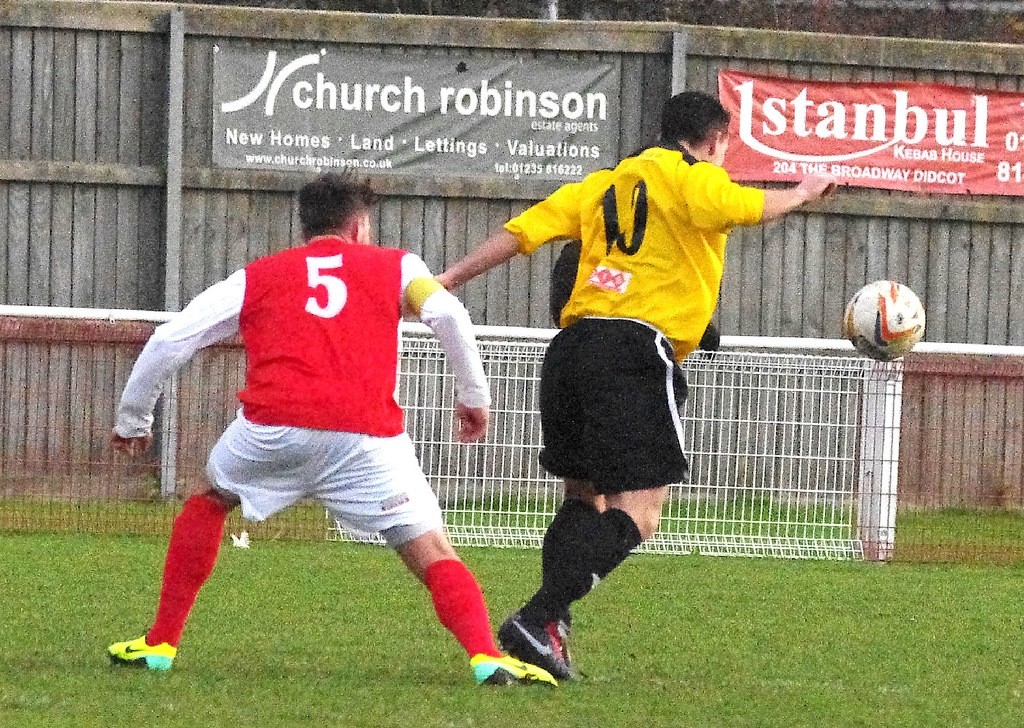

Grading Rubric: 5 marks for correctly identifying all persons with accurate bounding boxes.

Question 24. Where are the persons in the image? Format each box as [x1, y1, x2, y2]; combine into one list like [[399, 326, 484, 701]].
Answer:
[[107, 177, 559, 691], [436, 91, 837, 677]]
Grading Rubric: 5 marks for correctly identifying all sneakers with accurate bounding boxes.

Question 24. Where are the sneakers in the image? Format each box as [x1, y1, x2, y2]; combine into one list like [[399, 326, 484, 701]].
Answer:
[[108, 634, 178, 671], [498, 609, 573, 680], [470, 654, 556, 688]]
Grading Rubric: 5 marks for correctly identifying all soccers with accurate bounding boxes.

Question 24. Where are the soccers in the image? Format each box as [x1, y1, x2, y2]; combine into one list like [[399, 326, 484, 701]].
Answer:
[[845, 279, 927, 363]]
